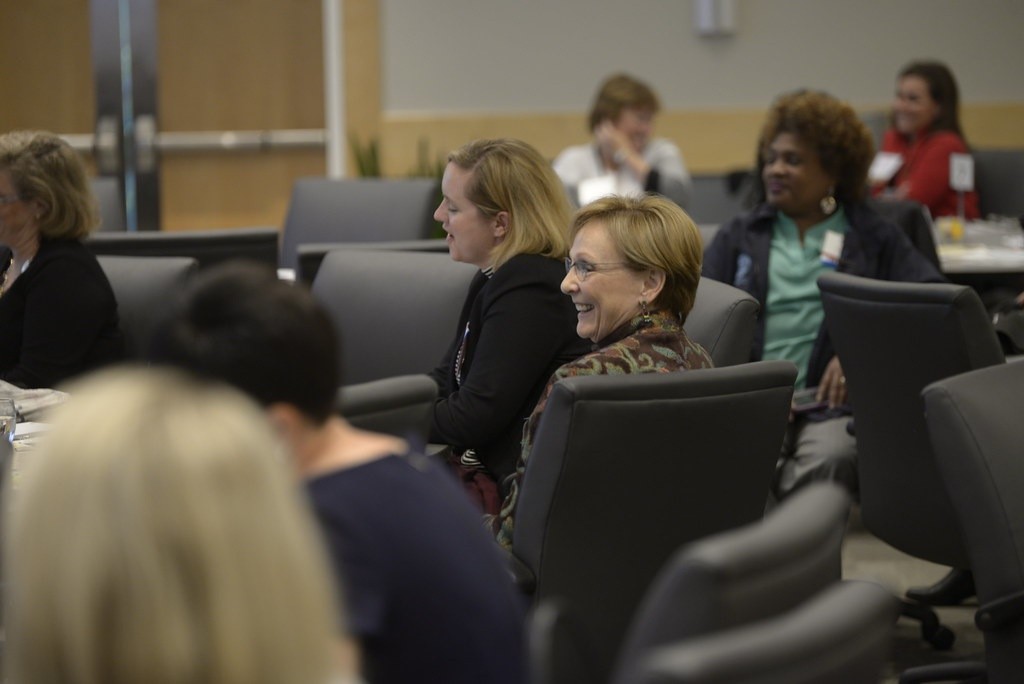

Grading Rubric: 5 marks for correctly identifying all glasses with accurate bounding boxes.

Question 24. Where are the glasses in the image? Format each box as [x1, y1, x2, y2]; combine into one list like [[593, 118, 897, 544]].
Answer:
[[565, 257, 634, 281]]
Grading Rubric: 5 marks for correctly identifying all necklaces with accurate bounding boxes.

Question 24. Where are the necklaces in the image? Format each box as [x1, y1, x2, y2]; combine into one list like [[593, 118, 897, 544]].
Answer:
[[0, 254, 37, 296]]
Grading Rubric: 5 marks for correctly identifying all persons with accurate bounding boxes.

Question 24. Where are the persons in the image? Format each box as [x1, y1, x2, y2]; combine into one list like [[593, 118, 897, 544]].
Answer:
[[1, 366, 359, 683], [552, 73, 692, 209], [477, 194, 714, 553], [870, 61, 980, 221], [702, 87, 944, 499], [429, 139, 574, 514], [0, 131, 120, 388], [148, 273, 530, 683]]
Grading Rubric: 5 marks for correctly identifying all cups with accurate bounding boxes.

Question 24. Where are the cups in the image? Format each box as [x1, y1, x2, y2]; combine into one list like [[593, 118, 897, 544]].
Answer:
[[0, 398, 17, 443]]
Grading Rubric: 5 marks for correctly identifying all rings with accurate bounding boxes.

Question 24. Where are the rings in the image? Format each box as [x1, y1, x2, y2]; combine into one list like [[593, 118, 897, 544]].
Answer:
[[839, 377, 847, 385]]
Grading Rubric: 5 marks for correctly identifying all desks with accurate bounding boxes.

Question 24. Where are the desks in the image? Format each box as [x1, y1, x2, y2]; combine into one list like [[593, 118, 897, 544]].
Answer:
[[928, 228, 1024, 273]]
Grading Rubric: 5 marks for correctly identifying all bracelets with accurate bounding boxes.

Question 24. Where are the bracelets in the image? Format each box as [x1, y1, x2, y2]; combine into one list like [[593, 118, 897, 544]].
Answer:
[[611, 146, 634, 166]]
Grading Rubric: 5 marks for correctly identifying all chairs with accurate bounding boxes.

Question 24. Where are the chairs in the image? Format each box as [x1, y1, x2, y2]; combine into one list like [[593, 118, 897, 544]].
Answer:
[[85, 145, 1024, 684]]
